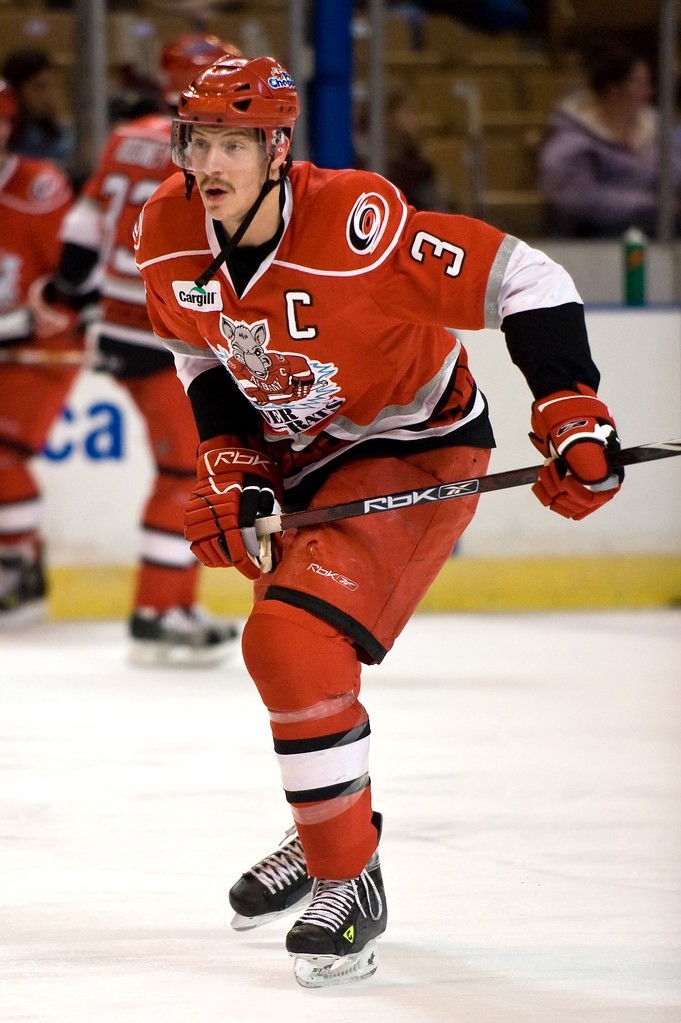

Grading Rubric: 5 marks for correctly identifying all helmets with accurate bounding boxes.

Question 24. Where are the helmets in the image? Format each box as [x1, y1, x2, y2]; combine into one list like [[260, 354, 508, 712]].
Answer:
[[171, 53, 299, 158], [0, 79, 19, 118], [160, 37, 242, 105]]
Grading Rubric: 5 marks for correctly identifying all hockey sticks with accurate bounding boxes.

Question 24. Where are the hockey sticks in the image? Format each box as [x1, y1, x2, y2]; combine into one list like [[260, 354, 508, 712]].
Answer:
[[255, 435, 680, 537]]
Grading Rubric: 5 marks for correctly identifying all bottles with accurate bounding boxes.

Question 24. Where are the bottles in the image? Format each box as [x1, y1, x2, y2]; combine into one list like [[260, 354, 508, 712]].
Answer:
[[623, 229, 644, 307]]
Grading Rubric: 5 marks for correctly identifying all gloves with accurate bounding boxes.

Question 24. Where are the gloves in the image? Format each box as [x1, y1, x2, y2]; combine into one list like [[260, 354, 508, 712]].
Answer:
[[182, 435, 286, 580], [528, 382, 625, 521], [31, 275, 77, 343]]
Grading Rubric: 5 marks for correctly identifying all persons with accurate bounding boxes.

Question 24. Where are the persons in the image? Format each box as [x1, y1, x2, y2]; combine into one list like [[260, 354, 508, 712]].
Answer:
[[536, 47, 681, 241], [134, 54, 625, 989], [2, 48, 80, 174], [0, 76, 89, 613], [27, 34, 246, 647], [352, 87, 438, 213]]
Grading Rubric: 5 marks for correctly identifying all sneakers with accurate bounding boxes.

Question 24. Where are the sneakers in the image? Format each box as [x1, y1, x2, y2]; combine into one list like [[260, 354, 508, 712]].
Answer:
[[228, 824, 312, 932], [0, 531, 48, 631], [285, 811, 388, 988], [129, 603, 238, 666]]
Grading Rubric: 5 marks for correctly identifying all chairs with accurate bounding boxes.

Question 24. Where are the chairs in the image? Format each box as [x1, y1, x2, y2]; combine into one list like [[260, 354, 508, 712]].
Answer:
[[0, 0, 566, 241]]
